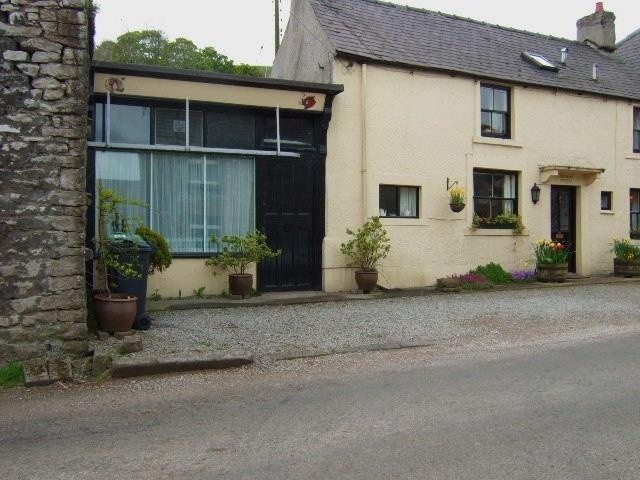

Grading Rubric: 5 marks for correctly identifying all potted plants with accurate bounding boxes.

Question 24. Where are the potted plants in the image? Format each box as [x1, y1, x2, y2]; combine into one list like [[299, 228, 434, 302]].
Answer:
[[205, 228, 282, 296], [340, 216, 390, 293], [88, 182, 171, 333]]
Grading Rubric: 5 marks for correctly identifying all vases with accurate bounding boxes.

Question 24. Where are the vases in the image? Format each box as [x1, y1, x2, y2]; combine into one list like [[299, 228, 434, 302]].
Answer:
[[536, 261, 570, 283], [450, 202, 465, 213], [613, 258, 640, 277]]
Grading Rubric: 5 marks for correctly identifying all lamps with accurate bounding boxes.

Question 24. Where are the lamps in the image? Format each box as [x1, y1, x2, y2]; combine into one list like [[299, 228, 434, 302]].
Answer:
[[531, 182, 541, 204]]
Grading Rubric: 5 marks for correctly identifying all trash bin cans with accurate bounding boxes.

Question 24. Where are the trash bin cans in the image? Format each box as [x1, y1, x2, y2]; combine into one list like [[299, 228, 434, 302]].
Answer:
[[106, 233, 153, 331]]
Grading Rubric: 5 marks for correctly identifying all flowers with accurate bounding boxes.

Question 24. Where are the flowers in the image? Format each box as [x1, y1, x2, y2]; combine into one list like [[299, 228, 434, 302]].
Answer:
[[607, 235, 640, 263], [449, 185, 466, 204], [531, 239, 571, 262]]
